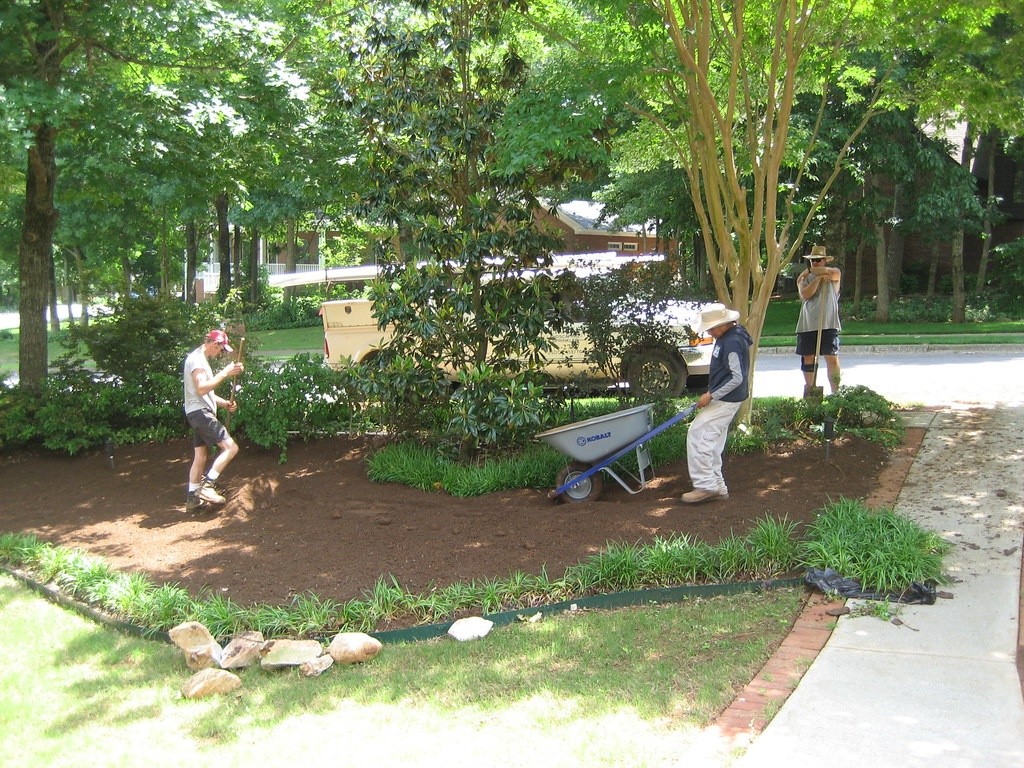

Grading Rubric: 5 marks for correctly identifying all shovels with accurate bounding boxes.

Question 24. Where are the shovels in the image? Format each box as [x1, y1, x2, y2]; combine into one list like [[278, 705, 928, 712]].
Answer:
[[225, 337, 247, 428], [802, 278, 825, 403]]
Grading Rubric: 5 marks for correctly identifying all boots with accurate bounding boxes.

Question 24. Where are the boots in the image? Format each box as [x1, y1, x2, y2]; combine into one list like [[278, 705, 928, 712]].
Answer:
[[193, 477, 227, 506], [186, 490, 212, 512]]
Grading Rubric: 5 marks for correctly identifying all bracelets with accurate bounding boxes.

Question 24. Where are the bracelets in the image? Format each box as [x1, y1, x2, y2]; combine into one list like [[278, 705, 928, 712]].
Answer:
[[808, 267, 811, 274]]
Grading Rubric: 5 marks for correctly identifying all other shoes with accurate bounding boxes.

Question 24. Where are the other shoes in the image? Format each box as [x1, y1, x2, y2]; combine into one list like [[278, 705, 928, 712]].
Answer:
[[682, 488, 717, 502], [702, 493, 729, 501]]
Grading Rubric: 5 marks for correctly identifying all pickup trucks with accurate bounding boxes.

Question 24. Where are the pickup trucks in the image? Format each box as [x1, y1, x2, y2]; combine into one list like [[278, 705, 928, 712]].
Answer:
[[315, 248, 739, 400]]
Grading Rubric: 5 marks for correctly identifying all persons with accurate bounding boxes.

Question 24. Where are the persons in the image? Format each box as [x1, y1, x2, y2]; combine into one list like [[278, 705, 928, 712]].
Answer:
[[797, 247, 843, 394], [184, 330, 244, 513], [680, 302, 753, 503]]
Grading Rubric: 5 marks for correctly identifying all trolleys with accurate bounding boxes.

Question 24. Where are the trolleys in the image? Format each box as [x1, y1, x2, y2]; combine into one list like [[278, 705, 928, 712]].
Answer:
[[532, 398, 699, 507]]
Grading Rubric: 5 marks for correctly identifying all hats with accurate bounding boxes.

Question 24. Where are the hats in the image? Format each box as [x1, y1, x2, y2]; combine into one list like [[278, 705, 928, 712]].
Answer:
[[206, 330, 233, 353], [802, 246, 834, 262], [690, 303, 740, 333]]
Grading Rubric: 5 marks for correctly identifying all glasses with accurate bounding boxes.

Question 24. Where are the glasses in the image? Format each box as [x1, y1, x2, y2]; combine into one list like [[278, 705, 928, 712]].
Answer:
[[809, 258, 821, 263]]
